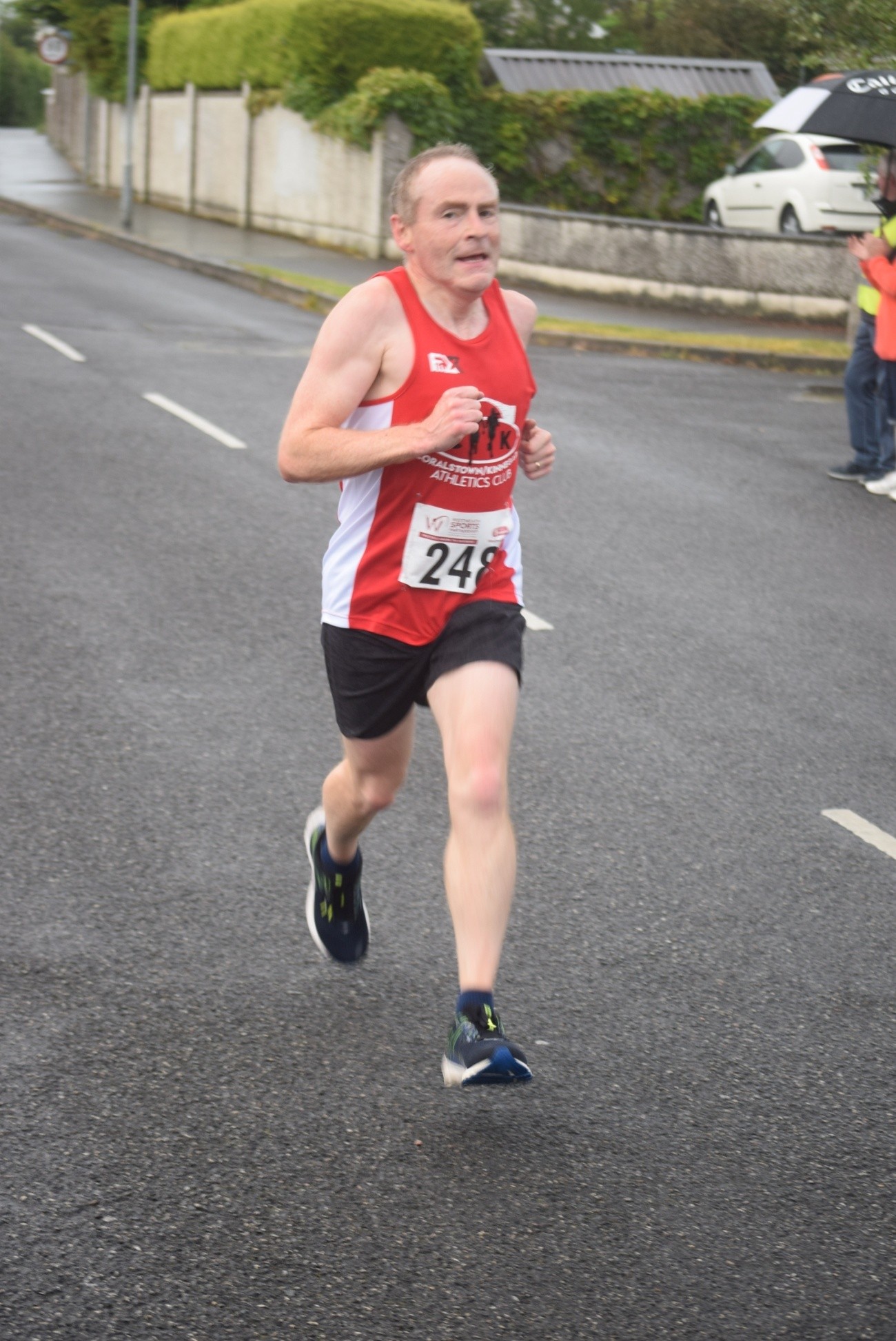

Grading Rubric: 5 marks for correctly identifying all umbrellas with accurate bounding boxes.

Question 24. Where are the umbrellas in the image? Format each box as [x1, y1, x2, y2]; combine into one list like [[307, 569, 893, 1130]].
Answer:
[[753, 67, 896, 219]]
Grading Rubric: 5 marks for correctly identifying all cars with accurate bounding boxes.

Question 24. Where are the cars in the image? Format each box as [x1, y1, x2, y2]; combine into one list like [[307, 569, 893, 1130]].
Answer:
[[699, 133, 884, 238]]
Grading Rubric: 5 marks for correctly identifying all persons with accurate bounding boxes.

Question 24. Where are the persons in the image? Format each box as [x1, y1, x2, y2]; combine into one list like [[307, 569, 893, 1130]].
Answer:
[[848, 231, 896, 501], [274, 146, 558, 1085], [826, 153, 896, 487]]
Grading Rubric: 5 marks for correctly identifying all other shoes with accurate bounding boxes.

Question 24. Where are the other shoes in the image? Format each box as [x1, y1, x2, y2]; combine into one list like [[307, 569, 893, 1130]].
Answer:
[[864, 471, 896, 494], [888, 489, 896, 501], [858, 474, 878, 485], [827, 462, 866, 480]]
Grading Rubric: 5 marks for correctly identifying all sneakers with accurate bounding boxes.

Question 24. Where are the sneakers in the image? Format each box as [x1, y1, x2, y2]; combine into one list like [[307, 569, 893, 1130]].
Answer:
[[442, 1003, 532, 1088], [303, 807, 372, 964]]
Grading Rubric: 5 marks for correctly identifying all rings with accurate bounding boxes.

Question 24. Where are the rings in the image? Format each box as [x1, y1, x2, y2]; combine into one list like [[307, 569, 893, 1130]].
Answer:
[[535, 461, 540, 471]]
